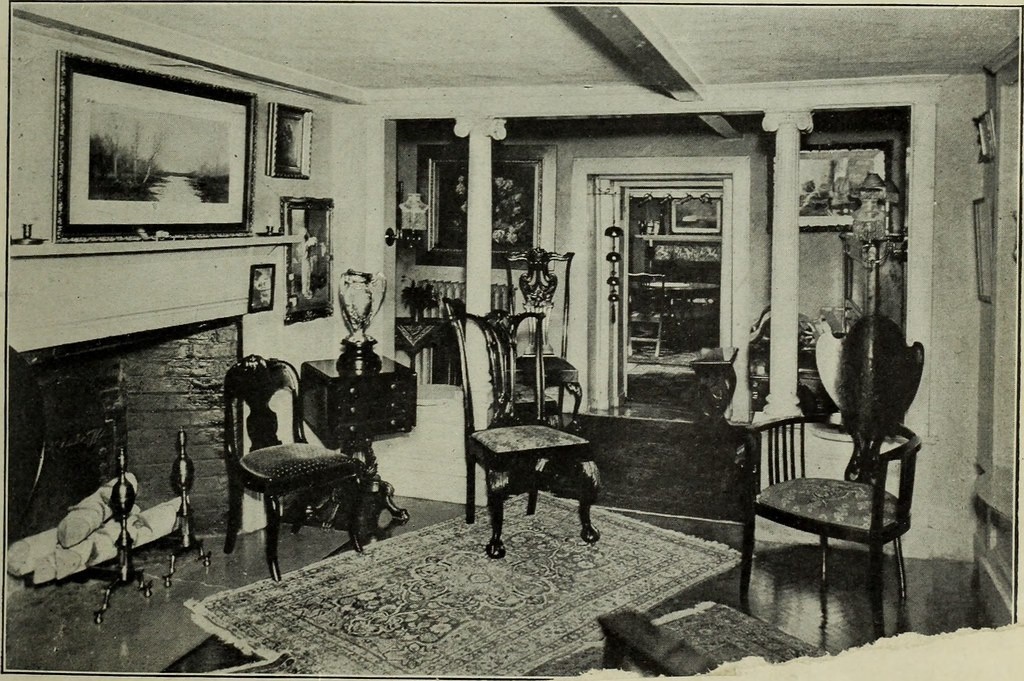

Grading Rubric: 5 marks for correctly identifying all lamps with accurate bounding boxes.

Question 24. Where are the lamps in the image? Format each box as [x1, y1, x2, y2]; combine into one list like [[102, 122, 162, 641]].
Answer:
[[386, 193, 428, 250]]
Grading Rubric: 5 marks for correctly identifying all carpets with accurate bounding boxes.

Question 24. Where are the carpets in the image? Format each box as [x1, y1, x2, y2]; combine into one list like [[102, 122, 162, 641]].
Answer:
[[185, 489, 754, 675], [526, 601, 825, 681]]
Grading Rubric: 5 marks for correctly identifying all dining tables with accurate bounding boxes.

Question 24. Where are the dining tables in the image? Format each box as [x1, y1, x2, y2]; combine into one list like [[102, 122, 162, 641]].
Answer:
[[642, 280, 719, 349]]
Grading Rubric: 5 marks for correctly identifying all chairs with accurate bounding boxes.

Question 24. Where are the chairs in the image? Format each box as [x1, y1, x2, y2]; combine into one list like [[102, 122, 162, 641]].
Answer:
[[627, 272, 672, 356], [740, 316, 924, 627], [443, 297, 599, 558], [224, 354, 368, 582], [500, 247, 582, 432]]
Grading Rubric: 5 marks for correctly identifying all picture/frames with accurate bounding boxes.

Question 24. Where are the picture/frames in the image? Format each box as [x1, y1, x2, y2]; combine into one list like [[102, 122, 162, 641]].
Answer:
[[280, 196, 334, 326], [766, 141, 894, 233], [671, 199, 721, 234], [972, 195, 995, 302], [265, 101, 314, 180], [973, 109, 998, 165], [50, 50, 258, 243], [248, 264, 276, 314], [416, 144, 556, 272]]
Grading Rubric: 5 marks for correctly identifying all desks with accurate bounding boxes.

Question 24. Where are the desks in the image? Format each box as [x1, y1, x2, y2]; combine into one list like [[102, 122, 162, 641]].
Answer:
[[396, 316, 453, 384], [302, 356, 418, 521]]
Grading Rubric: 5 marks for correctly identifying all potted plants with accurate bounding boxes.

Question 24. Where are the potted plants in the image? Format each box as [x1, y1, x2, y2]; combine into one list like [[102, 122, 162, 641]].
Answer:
[[402, 276, 438, 321]]
